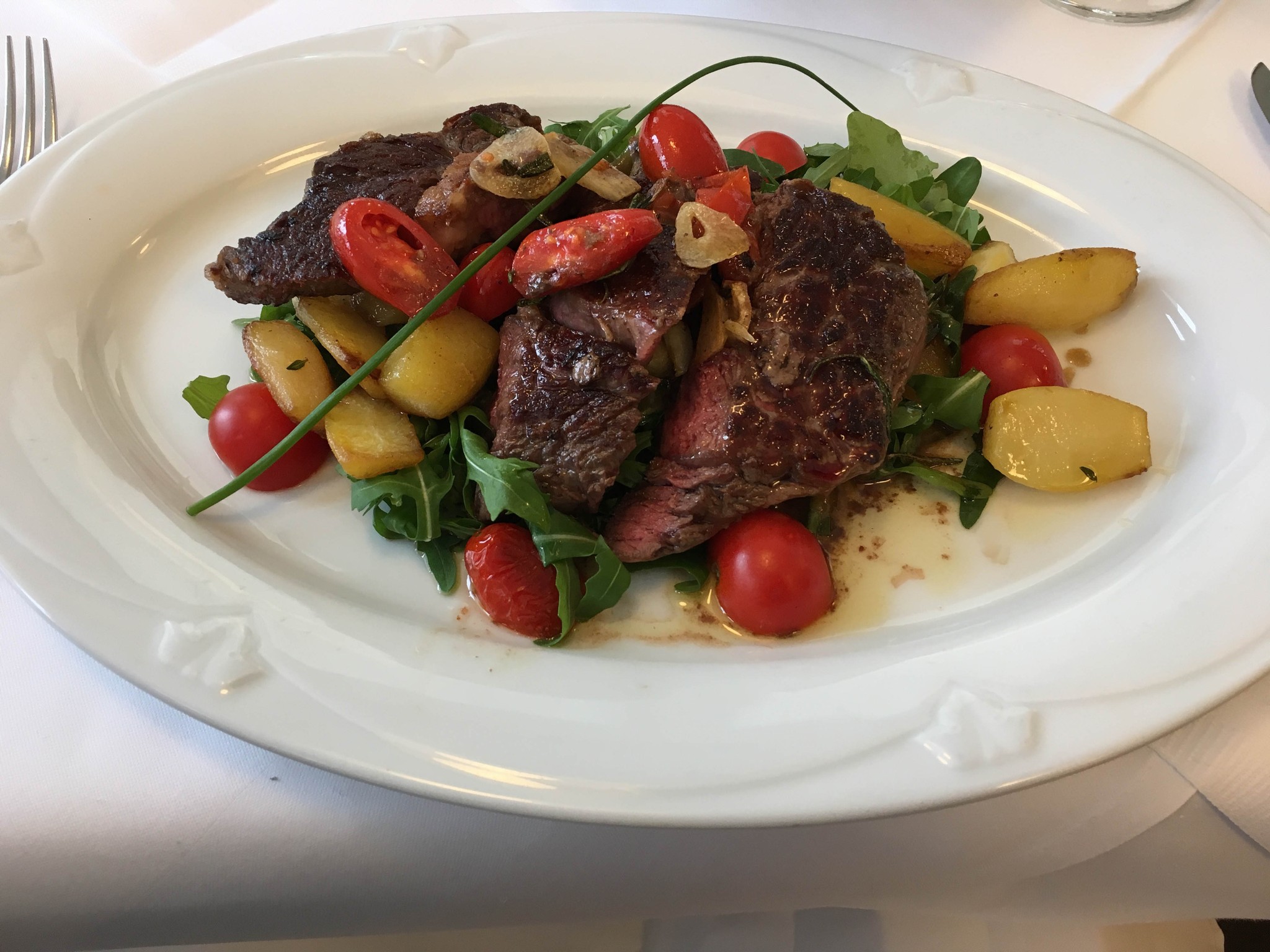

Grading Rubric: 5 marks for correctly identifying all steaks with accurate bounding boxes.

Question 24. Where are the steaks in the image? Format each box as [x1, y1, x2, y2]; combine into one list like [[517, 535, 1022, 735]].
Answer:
[[194, 97, 957, 582]]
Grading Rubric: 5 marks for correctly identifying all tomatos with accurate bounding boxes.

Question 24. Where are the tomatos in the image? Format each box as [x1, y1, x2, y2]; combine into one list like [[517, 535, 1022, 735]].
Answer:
[[739, 128, 817, 175], [957, 321, 1064, 407], [467, 521, 578, 638], [636, 104, 726, 182], [203, 377, 346, 498], [716, 512, 831, 636], [504, 205, 660, 294], [330, 193, 463, 319], [692, 168, 757, 236], [447, 236, 526, 324]]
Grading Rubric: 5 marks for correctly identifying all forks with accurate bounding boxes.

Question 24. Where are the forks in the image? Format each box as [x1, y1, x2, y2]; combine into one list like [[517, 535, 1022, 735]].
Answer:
[[0, 38, 63, 185]]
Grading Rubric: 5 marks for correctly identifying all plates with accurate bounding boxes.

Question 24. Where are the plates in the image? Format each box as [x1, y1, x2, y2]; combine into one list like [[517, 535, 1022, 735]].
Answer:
[[0, 6, 1268, 818]]
